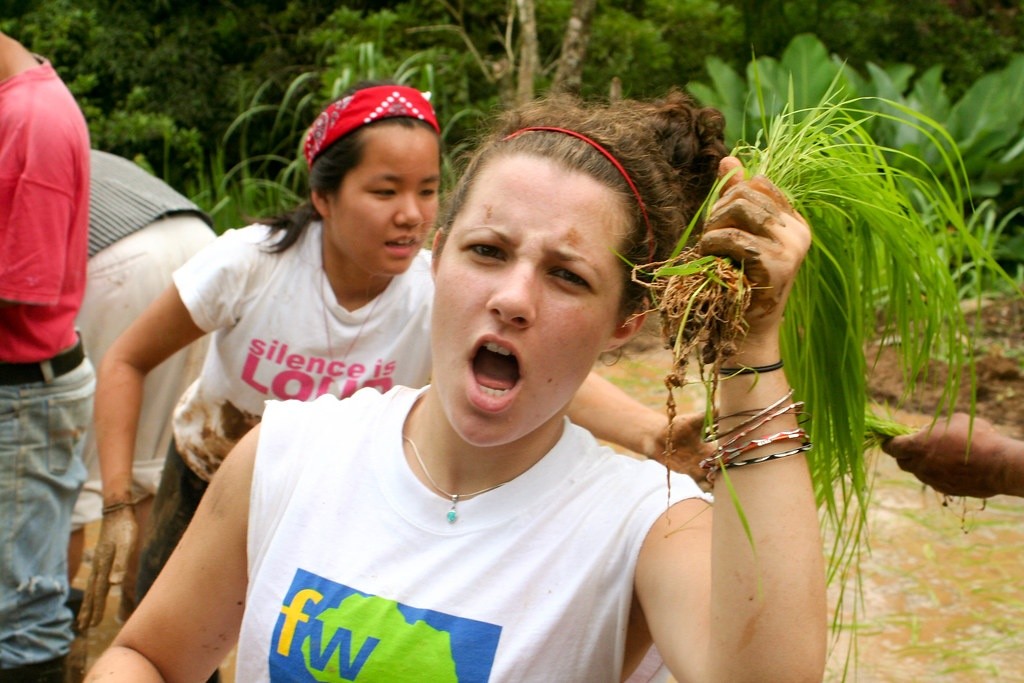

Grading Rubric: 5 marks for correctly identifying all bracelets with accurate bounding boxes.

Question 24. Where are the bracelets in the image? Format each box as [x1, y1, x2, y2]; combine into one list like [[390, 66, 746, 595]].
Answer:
[[103, 502, 135, 514], [700, 359, 814, 473]]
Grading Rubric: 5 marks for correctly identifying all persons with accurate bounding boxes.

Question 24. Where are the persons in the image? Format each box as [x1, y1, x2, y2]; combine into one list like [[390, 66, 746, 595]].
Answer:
[[86, 85, 827, 683], [66, 146, 222, 620], [2, 27, 98, 682], [78, 86, 718, 633]]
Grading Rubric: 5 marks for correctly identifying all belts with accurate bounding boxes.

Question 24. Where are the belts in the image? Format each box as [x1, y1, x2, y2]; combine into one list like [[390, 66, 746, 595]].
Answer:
[[0, 330, 84, 386]]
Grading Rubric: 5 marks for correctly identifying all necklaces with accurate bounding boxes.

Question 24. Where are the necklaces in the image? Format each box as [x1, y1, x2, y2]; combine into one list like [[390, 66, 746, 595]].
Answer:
[[400, 437, 511, 525]]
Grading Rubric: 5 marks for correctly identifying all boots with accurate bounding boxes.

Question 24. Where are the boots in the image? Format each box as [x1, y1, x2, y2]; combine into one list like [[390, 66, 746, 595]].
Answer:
[[0, 653, 67, 683]]
